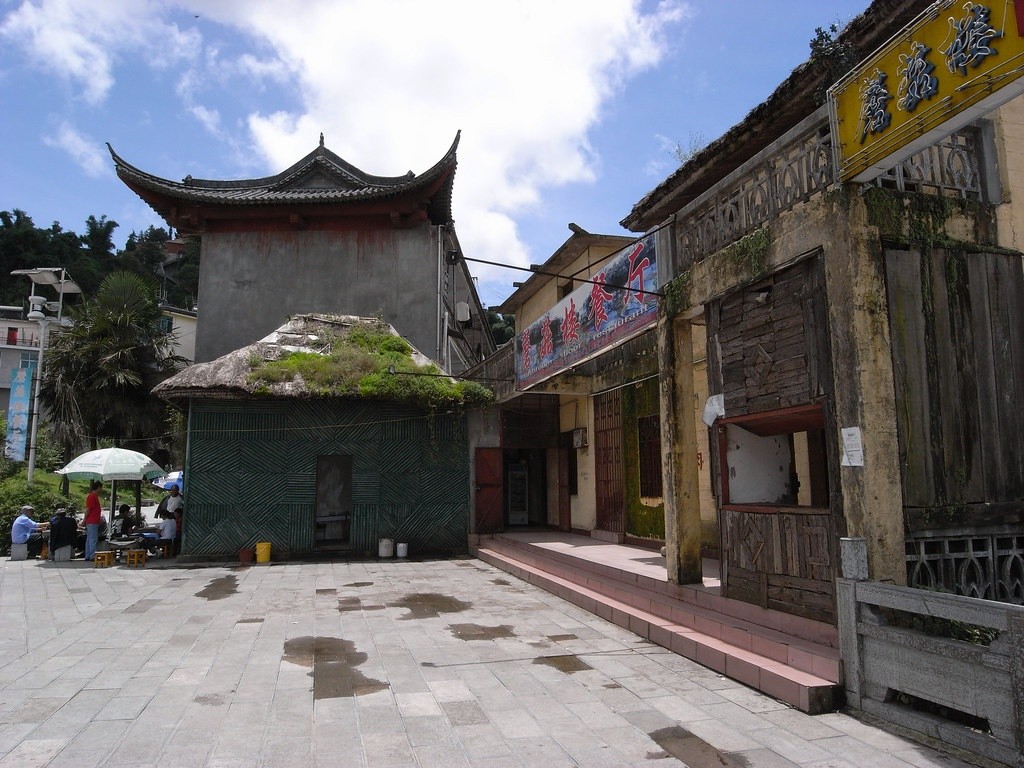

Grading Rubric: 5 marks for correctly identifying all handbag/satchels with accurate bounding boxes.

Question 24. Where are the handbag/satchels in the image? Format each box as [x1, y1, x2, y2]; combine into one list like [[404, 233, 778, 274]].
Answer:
[[41, 543, 48, 559]]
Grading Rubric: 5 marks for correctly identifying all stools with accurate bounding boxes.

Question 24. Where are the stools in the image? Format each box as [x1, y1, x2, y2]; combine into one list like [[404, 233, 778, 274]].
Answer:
[[156, 542, 173, 558], [127, 549, 147, 569], [94, 550, 115, 568]]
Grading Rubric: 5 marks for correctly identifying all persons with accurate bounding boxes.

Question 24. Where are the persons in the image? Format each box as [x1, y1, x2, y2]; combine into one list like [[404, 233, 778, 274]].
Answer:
[[82, 481, 103, 561], [11, 504, 50, 560], [154, 484, 184, 520]]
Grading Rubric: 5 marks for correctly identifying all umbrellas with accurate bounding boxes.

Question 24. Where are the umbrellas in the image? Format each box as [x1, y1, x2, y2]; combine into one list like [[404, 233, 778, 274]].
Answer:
[[152, 470, 184, 495], [54, 447, 169, 541], [50, 504, 184, 559]]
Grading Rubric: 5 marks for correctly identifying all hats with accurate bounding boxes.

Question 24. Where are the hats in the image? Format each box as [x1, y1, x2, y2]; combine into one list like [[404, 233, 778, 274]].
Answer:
[[56, 509, 66, 515], [22, 506, 34, 512]]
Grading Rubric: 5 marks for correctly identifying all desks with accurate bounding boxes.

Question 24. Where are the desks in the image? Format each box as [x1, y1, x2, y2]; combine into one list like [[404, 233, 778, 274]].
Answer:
[[42, 529, 85, 537], [128, 532, 161, 545]]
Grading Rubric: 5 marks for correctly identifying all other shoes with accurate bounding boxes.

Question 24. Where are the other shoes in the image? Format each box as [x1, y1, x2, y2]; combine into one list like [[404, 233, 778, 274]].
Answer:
[[27, 555, 39, 559]]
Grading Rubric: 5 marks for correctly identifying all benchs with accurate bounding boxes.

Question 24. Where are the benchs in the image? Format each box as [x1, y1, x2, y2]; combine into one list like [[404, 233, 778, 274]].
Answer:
[[110, 540, 139, 561], [142, 499, 155, 506]]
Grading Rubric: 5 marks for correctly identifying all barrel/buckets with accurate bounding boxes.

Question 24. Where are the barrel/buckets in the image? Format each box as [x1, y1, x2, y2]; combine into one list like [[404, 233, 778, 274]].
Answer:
[[240, 550, 254, 562], [379, 538, 394, 558], [396, 543, 407, 557], [254, 542, 271, 563]]
